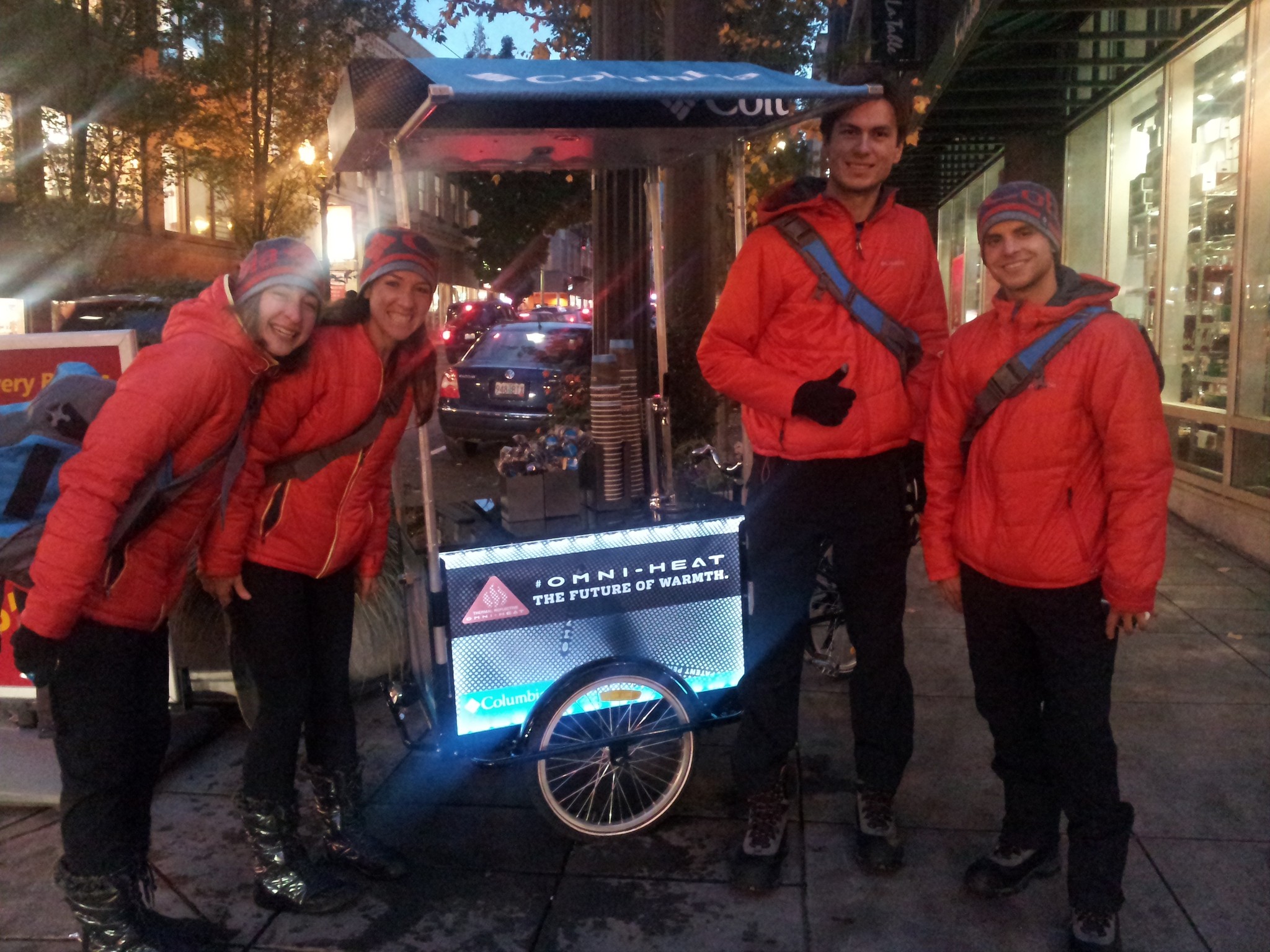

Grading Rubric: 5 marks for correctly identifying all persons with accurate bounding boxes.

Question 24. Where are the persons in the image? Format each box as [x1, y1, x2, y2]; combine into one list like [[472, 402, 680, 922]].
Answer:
[[695, 77, 954, 893], [920, 182, 1177, 952], [198, 226, 435, 913], [12, 236, 329, 952]]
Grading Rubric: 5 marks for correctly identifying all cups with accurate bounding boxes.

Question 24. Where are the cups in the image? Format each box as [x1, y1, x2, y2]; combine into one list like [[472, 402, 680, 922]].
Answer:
[[589, 338, 646, 511]]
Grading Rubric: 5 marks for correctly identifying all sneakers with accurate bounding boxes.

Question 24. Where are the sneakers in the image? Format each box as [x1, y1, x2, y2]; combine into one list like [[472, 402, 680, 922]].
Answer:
[[856, 787, 896, 853], [963, 835, 1062, 897], [1070, 904, 1124, 952], [741, 766, 790, 868]]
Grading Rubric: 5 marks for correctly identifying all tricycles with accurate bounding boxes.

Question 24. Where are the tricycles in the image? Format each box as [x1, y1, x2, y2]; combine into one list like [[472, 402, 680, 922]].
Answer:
[[392, 55, 862, 848]]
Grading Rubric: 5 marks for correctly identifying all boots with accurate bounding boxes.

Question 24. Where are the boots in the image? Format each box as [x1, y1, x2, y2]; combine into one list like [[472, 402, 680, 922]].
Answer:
[[302, 752, 406, 880], [54, 851, 198, 952], [237, 782, 351, 917]]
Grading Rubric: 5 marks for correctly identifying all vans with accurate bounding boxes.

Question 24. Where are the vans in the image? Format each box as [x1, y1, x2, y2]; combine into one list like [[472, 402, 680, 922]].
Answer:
[[443, 299, 518, 364]]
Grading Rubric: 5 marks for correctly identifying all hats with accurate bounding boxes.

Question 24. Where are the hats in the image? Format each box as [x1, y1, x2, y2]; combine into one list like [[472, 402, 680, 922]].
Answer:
[[357, 227, 440, 298], [977, 180, 1061, 261], [235, 235, 331, 311]]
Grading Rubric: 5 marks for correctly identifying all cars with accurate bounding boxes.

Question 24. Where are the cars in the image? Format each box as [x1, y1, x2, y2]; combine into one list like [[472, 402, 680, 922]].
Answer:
[[55, 295, 173, 350], [436, 308, 592, 455]]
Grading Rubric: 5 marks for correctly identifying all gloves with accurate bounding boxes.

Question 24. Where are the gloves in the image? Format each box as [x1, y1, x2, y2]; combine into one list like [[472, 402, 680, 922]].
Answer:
[[9, 624, 61, 689]]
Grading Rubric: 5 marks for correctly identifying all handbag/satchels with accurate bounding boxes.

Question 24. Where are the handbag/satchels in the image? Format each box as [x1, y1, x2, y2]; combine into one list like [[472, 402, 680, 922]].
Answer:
[[0, 362, 172, 590]]
